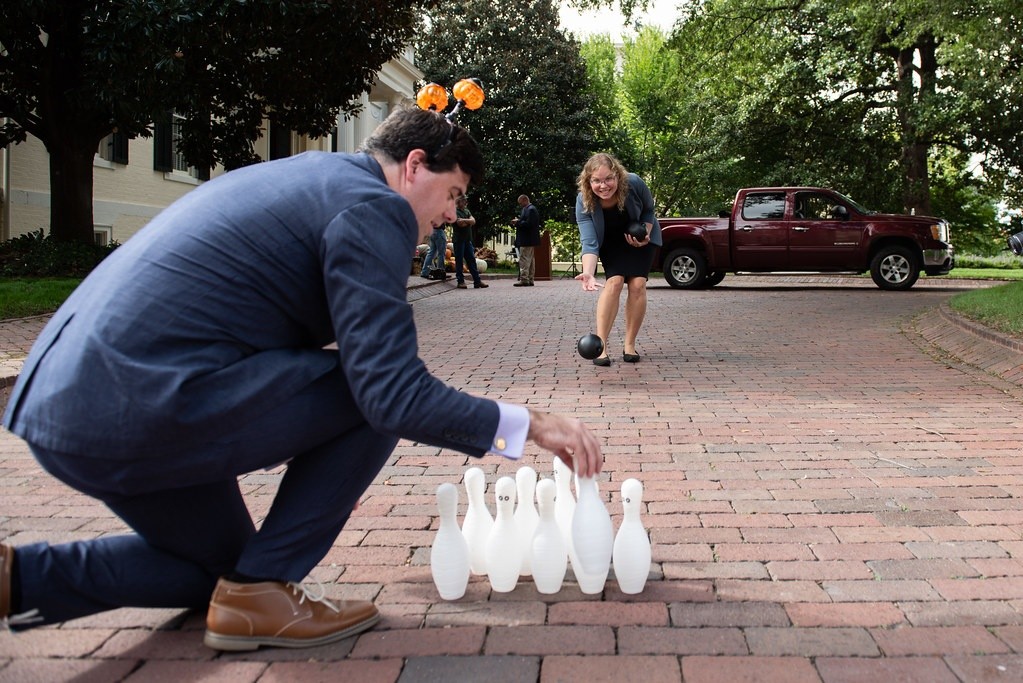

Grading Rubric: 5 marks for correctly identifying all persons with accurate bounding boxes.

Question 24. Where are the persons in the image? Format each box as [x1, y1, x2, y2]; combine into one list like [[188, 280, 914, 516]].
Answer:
[[511, 195, 539, 287], [420, 221, 451, 278], [0, 108, 602, 652], [574, 153, 662, 366], [453, 195, 489, 289]]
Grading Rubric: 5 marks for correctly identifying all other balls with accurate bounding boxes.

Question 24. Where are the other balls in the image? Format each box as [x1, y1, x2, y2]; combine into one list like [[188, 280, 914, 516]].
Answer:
[[627, 223, 646, 242], [576, 334, 605, 360]]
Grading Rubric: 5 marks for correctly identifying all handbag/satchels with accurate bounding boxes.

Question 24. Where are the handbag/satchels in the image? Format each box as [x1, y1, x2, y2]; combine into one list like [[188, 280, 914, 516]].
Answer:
[[429, 269, 446, 279]]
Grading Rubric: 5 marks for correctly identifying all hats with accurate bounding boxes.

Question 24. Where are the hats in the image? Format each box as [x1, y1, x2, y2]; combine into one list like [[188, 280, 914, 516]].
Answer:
[[455, 194, 468, 205]]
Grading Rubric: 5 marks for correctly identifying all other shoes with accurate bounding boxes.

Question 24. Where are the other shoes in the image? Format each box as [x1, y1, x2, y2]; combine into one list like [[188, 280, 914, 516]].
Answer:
[[514, 281, 530, 286], [623, 349, 640, 362], [474, 281, 489, 288], [530, 282, 534, 286], [593, 355, 610, 366], [457, 281, 467, 289]]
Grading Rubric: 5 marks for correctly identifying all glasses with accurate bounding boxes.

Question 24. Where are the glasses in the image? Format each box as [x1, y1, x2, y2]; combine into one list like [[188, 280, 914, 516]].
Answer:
[[589, 174, 617, 184]]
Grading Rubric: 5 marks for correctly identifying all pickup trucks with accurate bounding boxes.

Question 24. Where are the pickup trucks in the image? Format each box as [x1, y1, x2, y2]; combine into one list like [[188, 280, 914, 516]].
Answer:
[[656, 186, 955, 291]]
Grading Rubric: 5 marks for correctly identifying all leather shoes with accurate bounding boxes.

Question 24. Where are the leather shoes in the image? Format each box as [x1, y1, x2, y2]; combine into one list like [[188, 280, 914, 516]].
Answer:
[[0, 542, 12, 617], [204, 577, 381, 651]]
